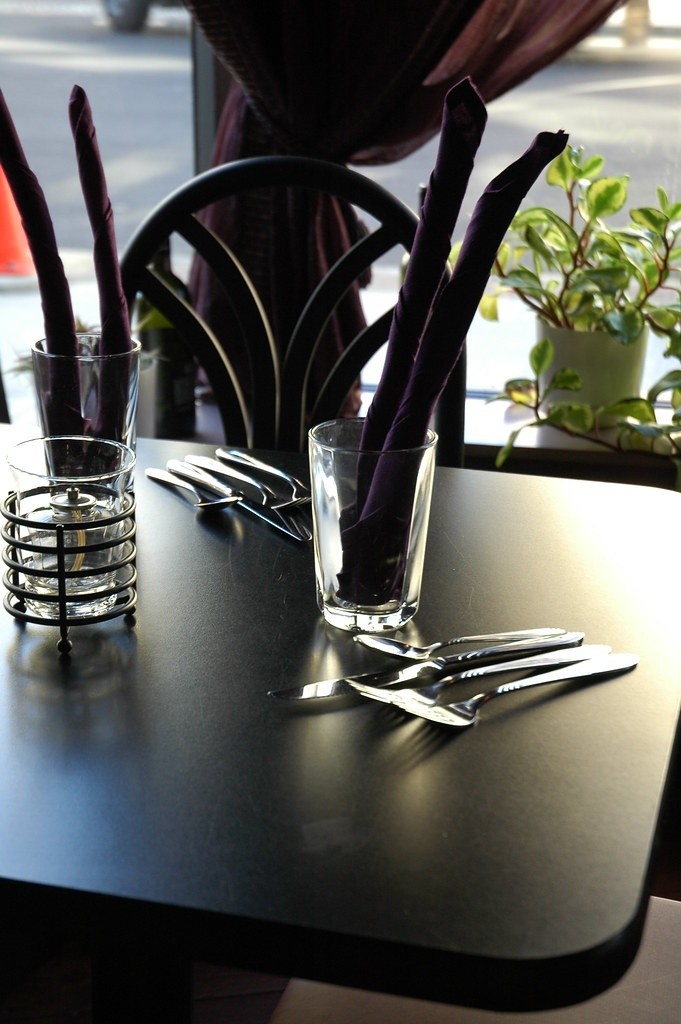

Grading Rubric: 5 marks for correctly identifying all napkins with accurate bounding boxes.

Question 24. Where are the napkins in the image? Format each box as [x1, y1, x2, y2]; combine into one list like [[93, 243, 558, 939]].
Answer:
[[0, 78, 131, 471], [338, 76, 570, 601]]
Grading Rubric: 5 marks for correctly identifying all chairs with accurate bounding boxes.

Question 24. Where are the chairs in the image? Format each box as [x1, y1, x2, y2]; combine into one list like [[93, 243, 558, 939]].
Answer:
[[92, 151, 468, 469]]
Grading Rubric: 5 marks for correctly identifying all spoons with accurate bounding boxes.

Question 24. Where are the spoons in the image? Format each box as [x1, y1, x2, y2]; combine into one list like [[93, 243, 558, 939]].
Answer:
[[145, 467, 243, 512], [353, 626, 567, 661]]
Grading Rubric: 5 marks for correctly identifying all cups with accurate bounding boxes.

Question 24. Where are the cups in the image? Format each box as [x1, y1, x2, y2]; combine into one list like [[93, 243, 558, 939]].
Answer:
[[306, 417, 439, 632], [31, 332, 141, 501], [6, 436, 138, 618]]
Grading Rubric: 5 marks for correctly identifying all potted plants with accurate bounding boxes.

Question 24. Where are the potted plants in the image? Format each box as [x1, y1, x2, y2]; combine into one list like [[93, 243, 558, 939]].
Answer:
[[447, 146, 681, 460]]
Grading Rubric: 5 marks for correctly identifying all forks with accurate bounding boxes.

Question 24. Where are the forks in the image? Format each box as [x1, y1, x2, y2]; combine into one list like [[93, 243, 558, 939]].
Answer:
[[345, 644, 613, 706], [392, 653, 640, 726], [184, 454, 311, 510]]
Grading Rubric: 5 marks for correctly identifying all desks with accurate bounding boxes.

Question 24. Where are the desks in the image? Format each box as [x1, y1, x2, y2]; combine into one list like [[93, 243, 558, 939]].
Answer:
[[0, 424, 681, 1024]]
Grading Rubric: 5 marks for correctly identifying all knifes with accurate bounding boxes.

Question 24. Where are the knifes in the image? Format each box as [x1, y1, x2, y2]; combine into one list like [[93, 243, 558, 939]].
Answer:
[[267, 631, 587, 701], [167, 458, 312, 542]]
